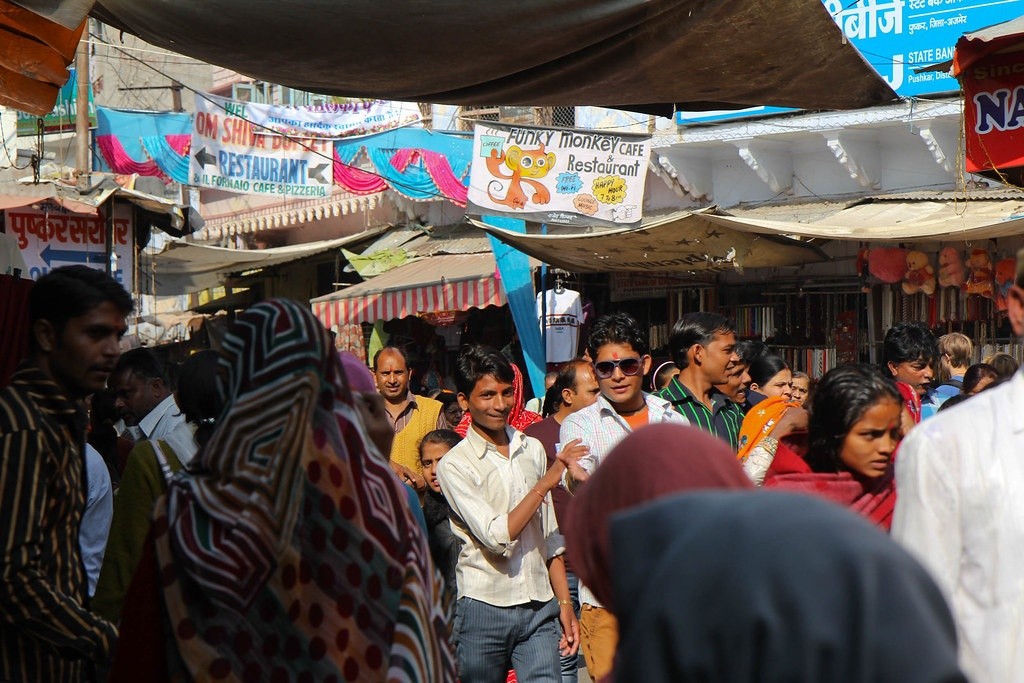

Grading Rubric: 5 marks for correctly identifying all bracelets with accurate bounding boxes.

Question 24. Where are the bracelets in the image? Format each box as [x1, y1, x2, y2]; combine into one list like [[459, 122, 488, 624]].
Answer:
[[560, 601, 572, 605], [533, 487, 544, 497], [765, 436, 778, 445], [757, 442, 776, 456], [760, 440, 778, 450], [566, 480, 576, 496]]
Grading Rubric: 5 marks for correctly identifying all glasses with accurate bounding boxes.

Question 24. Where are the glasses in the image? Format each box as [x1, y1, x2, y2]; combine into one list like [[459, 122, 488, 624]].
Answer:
[[592, 356, 644, 378], [936, 353, 945, 361]]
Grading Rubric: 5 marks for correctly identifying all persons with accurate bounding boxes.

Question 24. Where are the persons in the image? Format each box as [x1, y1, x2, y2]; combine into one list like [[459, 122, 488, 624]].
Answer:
[[602, 489, 968, 683], [526, 372, 557, 414], [767, 362, 903, 532], [882, 321, 1017, 421], [649, 312, 808, 484], [0, 266, 590, 682], [525, 358, 600, 683], [557, 315, 690, 683], [891, 248, 1024, 683]]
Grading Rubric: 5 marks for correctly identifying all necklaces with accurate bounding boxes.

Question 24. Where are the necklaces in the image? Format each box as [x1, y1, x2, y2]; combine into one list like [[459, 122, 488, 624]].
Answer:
[[616, 402, 646, 413]]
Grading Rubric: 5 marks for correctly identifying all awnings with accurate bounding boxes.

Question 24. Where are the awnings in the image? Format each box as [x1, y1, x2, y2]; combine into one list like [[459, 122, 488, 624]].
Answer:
[[310, 253, 513, 328], [690, 195, 1024, 239], [144, 217, 392, 261], [466, 206, 824, 278], [143, 310, 227, 328], [185, 285, 257, 313]]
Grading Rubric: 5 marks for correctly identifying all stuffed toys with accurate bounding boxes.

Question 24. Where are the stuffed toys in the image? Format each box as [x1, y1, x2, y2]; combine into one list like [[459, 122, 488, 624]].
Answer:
[[868, 247, 1017, 298]]
